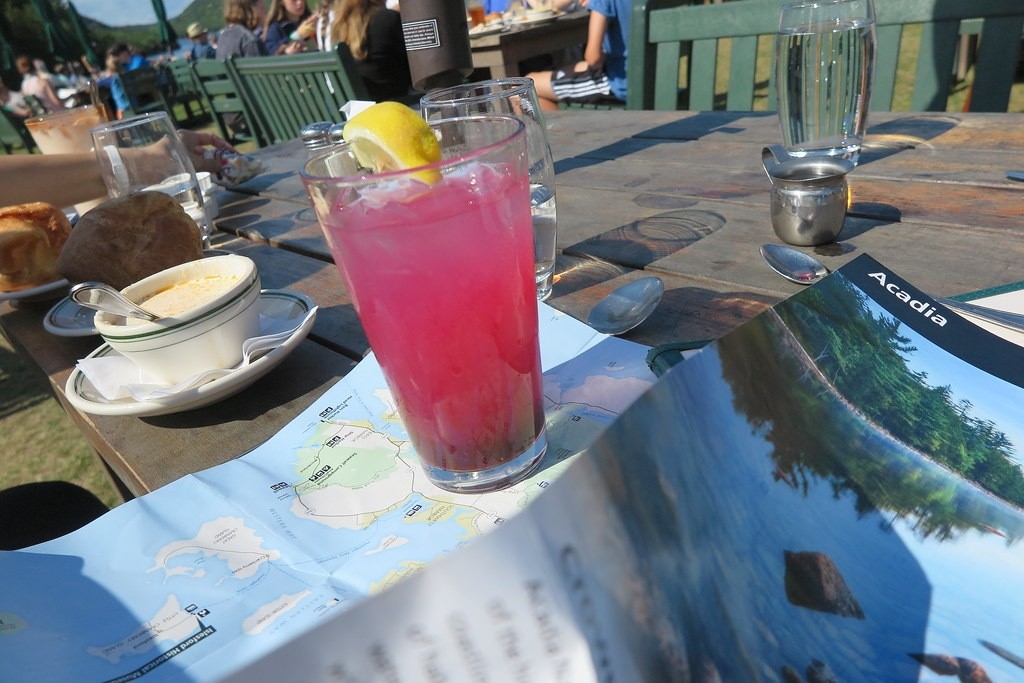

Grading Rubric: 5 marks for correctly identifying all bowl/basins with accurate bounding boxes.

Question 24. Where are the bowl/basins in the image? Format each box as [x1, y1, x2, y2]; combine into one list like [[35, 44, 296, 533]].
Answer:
[[93, 255, 262, 388], [526, 7, 553, 21]]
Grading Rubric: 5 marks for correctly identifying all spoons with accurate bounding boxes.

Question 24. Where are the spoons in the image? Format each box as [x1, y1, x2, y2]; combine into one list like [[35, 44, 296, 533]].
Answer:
[[760, 242, 828, 285], [586, 276, 664, 337]]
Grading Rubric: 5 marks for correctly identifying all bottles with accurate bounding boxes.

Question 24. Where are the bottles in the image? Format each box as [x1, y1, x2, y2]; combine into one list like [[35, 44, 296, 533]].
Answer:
[[399, 0, 474, 144], [301, 120, 335, 174], [328, 120, 363, 172]]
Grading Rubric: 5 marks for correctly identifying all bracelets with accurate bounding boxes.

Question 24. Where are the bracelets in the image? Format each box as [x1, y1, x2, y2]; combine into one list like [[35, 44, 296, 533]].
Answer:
[[91, 144, 129, 199]]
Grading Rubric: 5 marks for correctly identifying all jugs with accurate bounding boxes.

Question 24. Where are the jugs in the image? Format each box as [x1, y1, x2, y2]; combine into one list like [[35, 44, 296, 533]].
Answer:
[[760, 143, 855, 246]]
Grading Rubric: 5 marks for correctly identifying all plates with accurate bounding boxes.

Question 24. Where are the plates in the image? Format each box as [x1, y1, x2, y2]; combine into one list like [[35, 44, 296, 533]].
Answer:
[[42, 294, 100, 337], [469, 27, 506, 38], [64, 288, 317, 418], [0, 279, 69, 300], [512, 12, 566, 25]]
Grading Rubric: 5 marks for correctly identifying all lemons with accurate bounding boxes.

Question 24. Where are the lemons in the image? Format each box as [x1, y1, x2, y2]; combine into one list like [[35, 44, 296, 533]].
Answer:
[[341, 101, 445, 186]]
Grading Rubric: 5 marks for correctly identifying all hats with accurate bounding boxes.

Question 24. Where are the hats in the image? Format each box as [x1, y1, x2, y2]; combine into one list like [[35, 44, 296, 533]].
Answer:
[[187, 23, 208, 38]]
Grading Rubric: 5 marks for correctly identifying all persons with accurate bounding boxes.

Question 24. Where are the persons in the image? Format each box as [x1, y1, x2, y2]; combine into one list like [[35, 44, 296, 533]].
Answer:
[[0, 129, 239, 211], [0, 42, 153, 117], [186, 22, 217, 61], [215, 0, 424, 136], [482, 0, 632, 116]]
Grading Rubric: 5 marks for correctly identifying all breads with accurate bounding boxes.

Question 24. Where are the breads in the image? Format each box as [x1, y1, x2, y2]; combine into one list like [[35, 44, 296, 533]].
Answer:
[[0, 200, 75, 294], [482, 12, 501, 23], [56, 191, 203, 301]]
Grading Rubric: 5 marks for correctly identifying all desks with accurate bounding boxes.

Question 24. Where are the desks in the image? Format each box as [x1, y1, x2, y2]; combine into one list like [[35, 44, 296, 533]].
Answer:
[[469, 11, 593, 95], [0, 110, 1024, 502]]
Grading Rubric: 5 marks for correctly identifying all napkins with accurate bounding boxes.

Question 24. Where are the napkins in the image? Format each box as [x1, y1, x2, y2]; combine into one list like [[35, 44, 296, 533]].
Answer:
[[74, 309, 318, 403]]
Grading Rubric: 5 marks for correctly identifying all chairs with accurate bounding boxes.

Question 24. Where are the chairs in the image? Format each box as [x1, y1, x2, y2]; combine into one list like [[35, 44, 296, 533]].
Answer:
[[23, 94, 50, 117], [624, 0, 1024, 112], [0, 105, 37, 154], [89, 42, 372, 149]]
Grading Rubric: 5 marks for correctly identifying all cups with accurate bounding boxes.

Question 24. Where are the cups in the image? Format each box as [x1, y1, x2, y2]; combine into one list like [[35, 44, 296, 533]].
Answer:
[[89, 111, 212, 239], [775, 0, 878, 165], [299, 116, 549, 493], [467, 3, 484, 27], [162, 170, 219, 219], [24, 102, 116, 219], [418, 77, 558, 301]]
